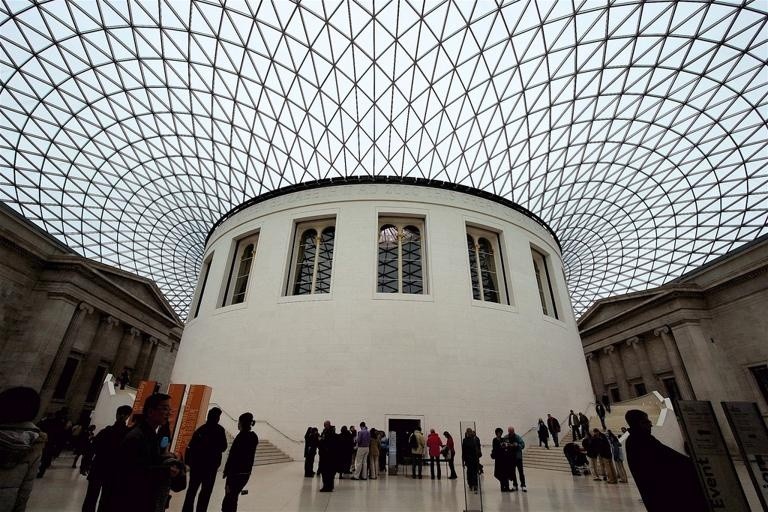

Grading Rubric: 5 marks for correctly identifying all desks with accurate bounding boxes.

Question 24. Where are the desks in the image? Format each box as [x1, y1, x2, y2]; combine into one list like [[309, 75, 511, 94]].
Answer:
[[399, 456, 451, 478]]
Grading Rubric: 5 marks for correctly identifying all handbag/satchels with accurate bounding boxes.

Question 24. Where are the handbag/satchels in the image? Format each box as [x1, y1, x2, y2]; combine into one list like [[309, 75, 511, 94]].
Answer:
[[441, 446, 452, 461]]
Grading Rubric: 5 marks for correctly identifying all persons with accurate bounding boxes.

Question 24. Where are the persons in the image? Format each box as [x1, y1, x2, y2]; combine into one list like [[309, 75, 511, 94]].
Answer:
[[180, 405, 228, 511], [0, 366, 185, 511], [601, 391, 611, 413], [220, 410, 260, 511], [624, 408, 711, 511], [595, 400, 606, 431]]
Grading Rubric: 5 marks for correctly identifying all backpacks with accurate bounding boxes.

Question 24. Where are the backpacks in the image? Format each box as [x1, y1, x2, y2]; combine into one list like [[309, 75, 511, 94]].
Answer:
[[409, 432, 418, 449]]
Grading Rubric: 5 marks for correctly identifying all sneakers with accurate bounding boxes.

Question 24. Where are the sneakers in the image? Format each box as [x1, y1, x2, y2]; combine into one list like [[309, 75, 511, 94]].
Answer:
[[469, 484, 527, 494], [304, 472, 378, 492], [572, 469, 628, 484], [411, 473, 457, 480]]
